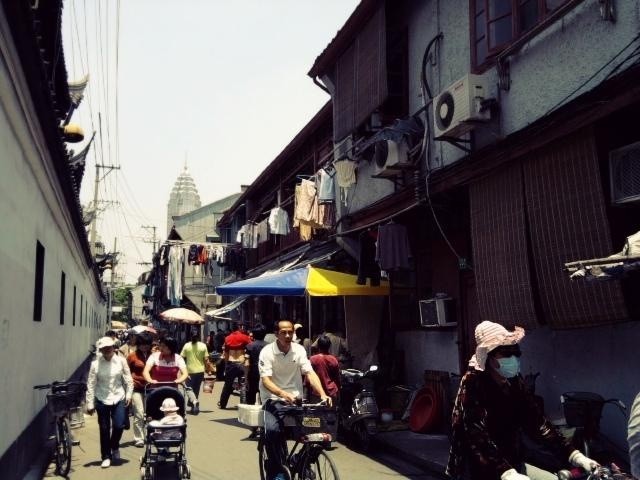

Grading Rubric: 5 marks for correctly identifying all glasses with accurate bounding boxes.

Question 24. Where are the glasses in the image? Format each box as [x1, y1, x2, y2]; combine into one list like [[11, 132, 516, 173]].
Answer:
[[499, 348, 523, 358]]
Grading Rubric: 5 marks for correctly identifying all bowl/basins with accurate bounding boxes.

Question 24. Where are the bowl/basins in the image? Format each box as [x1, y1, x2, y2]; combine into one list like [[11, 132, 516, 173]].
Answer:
[[411, 394, 433, 429], [408, 387, 437, 431]]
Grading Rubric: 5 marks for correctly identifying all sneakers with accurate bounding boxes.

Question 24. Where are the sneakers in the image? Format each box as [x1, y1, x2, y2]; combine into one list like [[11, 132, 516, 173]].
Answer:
[[217, 400, 222, 408], [113, 448, 120, 460], [135, 439, 144, 447], [291, 453, 316, 479], [101, 459, 110, 468], [248, 426, 263, 438], [274, 469, 290, 480], [190, 399, 199, 415]]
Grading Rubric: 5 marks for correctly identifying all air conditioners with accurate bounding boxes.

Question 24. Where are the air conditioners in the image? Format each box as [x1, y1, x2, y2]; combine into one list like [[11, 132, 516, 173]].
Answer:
[[608, 141, 640, 205], [371, 117, 409, 172], [432, 71, 492, 139], [420, 289, 459, 329], [204, 292, 223, 306]]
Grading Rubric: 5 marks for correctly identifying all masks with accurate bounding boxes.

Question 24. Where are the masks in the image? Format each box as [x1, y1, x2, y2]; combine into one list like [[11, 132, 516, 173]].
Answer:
[[493, 355, 522, 378]]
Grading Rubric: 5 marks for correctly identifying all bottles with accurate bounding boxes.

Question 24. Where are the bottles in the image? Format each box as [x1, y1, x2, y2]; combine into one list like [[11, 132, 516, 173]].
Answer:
[[304, 433, 332, 442]]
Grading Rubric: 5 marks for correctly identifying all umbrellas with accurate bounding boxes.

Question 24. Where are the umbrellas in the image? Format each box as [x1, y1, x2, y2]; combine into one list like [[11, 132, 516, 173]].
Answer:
[[215, 264, 390, 357], [159, 306, 206, 345]]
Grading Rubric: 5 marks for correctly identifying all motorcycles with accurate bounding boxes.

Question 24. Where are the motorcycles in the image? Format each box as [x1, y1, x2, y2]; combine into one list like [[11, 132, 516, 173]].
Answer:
[[340, 363, 385, 453]]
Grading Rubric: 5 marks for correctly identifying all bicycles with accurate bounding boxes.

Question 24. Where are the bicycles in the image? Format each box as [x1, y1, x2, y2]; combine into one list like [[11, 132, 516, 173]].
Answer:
[[558, 389, 627, 466], [249, 392, 341, 480], [544, 459, 639, 479], [32, 379, 89, 477]]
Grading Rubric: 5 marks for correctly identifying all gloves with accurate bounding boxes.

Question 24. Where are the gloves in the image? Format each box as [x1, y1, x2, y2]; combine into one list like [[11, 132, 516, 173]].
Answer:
[[500, 468, 531, 480], [568, 449, 602, 473]]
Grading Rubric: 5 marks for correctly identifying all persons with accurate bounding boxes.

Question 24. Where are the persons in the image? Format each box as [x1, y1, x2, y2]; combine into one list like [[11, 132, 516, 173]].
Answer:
[[86, 319, 341, 480], [445, 320, 603, 480], [627, 392, 640, 479]]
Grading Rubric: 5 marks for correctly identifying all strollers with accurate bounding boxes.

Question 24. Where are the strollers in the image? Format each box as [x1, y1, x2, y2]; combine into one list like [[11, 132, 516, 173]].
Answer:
[[140, 381, 195, 480]]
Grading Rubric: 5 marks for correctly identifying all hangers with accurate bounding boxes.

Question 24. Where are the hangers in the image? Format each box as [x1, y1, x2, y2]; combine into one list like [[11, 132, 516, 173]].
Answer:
[[295, 150, 357, 181]]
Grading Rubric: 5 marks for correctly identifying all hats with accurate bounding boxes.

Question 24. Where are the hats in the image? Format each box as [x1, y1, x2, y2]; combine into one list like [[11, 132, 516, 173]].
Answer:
[[159, 397, 180, 412], [467, 320, 526, 373], [95, 336, 116, 349]]
